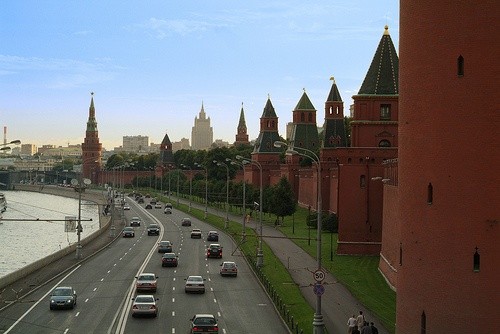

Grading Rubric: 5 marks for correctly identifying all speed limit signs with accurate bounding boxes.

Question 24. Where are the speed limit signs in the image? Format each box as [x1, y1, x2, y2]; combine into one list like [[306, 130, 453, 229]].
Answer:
[[313, 270, 325, 282]]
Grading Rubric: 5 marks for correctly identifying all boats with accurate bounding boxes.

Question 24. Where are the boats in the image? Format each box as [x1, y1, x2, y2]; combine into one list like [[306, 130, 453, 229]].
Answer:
[[0, 193, 7, 213]]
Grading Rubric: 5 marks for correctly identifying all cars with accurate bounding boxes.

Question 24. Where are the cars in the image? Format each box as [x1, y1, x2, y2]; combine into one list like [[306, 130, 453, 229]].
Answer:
[[189, 314, 219, 334], [130, 217, 141, 226], [135, 273, 158, 292], [184, 276, 206, 293], [161, 253, 178, 267], [131, 295, 159, 318], [123, 227, 134, 237], [117, 190, 173, 214], [181, 217, 238, 277], [147, 223, 160, 235], [50, 286, 77, 310]]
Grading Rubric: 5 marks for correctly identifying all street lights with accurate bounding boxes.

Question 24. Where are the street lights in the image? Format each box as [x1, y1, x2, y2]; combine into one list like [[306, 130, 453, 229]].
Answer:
[[235, 156, 263, 267], [273, 140, 325, 334], [226, 158, 246, 243], [101, 165, 180, 208], [213, 160, 229, 228], [194, 163, 208, 221], [181, 164, 192, 213]]
